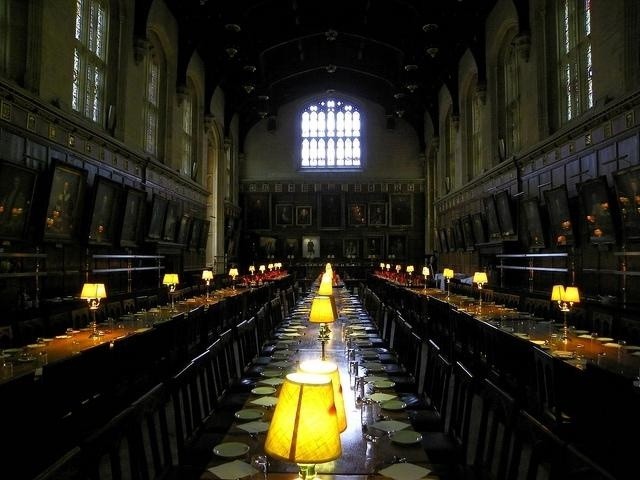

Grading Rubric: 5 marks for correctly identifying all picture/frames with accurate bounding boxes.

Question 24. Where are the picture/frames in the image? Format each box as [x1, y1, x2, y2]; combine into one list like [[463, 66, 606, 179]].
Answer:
[[433, 165, 640, 252], [243, 192, 414, 262], [0, 156, 211, 254]]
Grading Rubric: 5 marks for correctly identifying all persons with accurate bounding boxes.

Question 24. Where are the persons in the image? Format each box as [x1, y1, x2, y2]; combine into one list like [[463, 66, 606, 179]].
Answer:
[[278, 207, 292, 224], [2, 175, 26, 231], [298, 207, 311, 225], [388, 238, 404, 256], [90, 188, 190, 243], [344, 240, 357, 256], [367, 238, 379, 256], [306, 240, 317, 256], [47, 178, 74, 226], [368, 207, 385, 225]]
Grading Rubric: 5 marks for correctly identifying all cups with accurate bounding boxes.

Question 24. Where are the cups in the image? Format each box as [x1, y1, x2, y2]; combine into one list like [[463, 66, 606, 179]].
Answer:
[[1, 304, 171, 379], [345, 326, 365, 402], [455, 301, 626, 368]]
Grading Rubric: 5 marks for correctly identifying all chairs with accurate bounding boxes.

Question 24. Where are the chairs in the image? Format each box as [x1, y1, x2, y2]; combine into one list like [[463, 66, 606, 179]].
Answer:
[[0, 271, 640, 480]]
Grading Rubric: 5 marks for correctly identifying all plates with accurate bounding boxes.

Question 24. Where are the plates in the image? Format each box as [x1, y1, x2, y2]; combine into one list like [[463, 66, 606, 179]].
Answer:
[[360, 363, 424, 445], [213, 322, 306, 459], [621, 345, 640, 355]]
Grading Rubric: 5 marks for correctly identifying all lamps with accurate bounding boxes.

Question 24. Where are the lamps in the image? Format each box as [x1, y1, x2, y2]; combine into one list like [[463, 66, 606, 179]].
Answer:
[[202, 271, 214, 285], [263, 357, 349, 476], [162, 274, 180, 293], [472, 272, 488, 290], [551, 285, 580, 311], [80, 283, 107, 310], [317, 275, 333, 295], [443, 268, 454, 279], [308, 295, 335, 343]]
